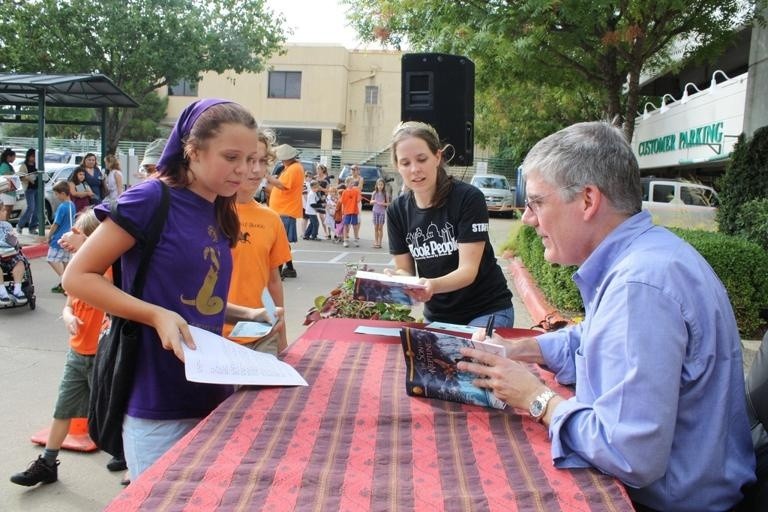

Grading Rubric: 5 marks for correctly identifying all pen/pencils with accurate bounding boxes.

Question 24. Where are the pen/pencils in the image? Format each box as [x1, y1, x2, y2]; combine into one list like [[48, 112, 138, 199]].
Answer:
[[485, 315, 495, 339]]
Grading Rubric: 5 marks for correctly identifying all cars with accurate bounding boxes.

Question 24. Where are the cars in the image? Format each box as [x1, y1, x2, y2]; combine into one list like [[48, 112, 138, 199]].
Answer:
[[252, 174, 269, 202], [468, 173, 514, 219], [0, 143, 102, 228]]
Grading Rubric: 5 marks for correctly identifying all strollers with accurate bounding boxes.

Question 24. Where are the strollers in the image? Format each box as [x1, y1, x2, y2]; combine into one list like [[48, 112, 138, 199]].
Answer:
[[0, 237, 37, 313]]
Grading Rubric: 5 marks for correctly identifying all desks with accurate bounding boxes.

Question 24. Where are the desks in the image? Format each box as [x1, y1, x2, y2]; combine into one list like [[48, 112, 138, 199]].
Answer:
[[105, 313, 643, 512]]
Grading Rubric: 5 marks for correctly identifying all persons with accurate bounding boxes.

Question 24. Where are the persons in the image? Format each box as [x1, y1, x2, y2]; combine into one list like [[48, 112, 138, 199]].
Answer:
[[219, 121, 294, 391], [453, 118, 763, 512], [6, 208, 131, 487], [386, 117, 514, 329], [59, 97, 286, 482]]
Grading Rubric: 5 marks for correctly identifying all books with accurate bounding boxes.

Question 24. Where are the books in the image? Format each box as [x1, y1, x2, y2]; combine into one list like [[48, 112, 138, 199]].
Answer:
[[347, 269, 426, 307], [175, 320, 310, 394], [399, 324, 518, 410]]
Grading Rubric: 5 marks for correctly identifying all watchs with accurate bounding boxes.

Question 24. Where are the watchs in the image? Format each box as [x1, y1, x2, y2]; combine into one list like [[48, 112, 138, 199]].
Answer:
[[527, 390, 560, 424]]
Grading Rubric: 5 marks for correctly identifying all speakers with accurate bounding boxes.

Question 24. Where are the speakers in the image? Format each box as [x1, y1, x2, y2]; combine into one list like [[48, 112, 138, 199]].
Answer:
[[401, 53, 474, 167]]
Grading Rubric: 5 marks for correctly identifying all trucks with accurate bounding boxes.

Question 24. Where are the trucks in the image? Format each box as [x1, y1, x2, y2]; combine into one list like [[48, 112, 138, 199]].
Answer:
[[514, 165, 525, 216]]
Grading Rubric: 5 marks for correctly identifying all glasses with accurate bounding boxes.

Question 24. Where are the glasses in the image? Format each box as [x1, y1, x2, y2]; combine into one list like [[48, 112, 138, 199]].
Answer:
[[525, 182, 583, 209]]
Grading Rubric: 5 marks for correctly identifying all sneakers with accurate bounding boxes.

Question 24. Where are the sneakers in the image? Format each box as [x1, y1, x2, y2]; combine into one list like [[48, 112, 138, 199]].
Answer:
[[10, 454, 62, 488], [303, 233, 382, 249], [51, 284, 63, 294], [283, 268, 297, 278], [87, 329, 140, 461], [29, 229, 39, 235], [16, 224, 24, 234], [0, 291, 27, 304]]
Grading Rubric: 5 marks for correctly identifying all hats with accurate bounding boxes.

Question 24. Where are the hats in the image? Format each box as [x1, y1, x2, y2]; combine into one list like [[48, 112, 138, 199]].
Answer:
[[275, 143, 302, 162], [139, 138, 169, 172]]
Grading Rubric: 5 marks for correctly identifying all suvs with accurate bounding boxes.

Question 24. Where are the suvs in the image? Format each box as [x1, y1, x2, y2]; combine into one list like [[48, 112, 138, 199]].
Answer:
[[336, 162, 395, 210], [271, 158, 328, 210], [637, 175, 724, 235]]
